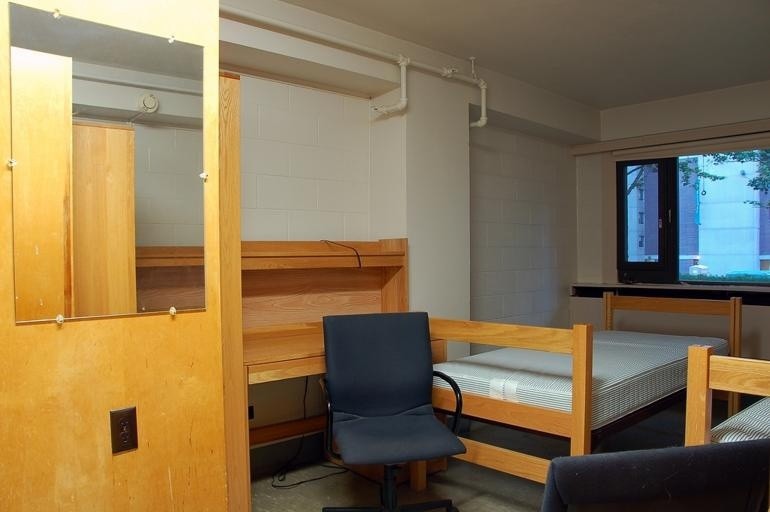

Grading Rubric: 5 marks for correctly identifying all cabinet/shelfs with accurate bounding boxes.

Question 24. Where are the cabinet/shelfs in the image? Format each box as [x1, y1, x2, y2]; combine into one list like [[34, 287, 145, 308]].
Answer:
[[240, 238, 408, 469]]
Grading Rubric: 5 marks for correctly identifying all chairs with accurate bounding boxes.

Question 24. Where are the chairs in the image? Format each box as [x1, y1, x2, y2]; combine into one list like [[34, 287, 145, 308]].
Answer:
[[319, 312, 466, 512], [541, 440, 770, 512]]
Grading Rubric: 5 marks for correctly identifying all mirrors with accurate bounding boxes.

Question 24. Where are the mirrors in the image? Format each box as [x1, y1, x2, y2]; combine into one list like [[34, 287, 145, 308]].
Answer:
[[8, 0, 208, 325]]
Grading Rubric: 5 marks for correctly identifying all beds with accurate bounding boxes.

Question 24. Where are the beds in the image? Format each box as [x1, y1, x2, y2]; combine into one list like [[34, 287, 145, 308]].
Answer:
[[425, 292, 744, 484], [684, 344, 770, 447]]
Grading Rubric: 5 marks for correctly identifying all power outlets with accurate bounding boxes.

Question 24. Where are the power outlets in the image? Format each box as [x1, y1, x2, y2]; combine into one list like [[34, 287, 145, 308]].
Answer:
[[248, 400, 257, 421], [111, 407, 139, 455]]
